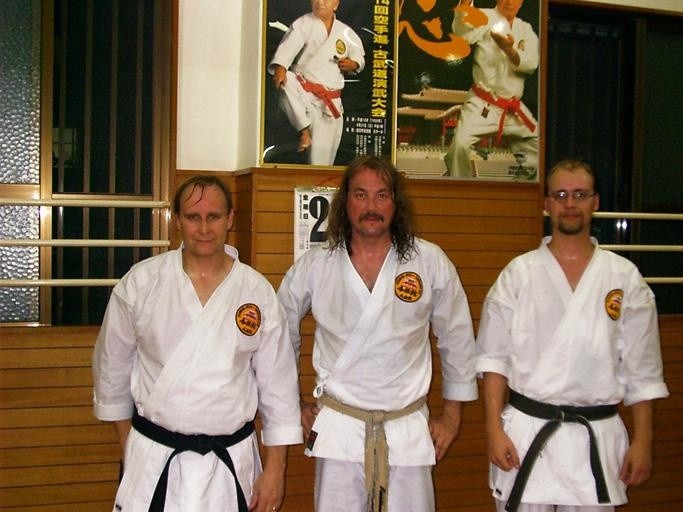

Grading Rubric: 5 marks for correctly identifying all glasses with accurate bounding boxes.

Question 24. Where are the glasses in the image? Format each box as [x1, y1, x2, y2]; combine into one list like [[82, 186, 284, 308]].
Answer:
[[551, 190, 596, 201]]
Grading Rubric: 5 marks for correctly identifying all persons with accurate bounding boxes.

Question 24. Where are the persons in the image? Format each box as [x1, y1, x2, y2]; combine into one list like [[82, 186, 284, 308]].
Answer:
[[278, 154, 480, 512], [475, 160, 671, 512], [267, 0, 365, 166], [92, 176, 304, 512], [444, 0, 540, 180]]
